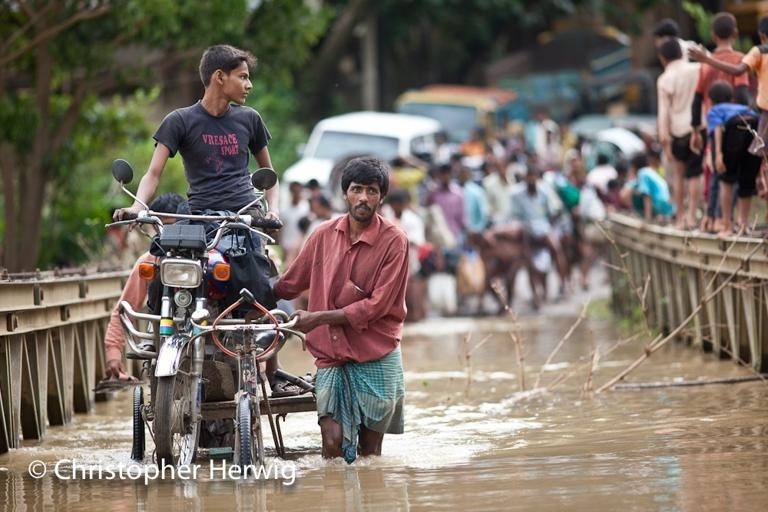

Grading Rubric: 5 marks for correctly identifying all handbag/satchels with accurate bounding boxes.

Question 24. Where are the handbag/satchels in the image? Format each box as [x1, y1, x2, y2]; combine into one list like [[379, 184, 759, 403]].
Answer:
[[228, 224, 278, 310]]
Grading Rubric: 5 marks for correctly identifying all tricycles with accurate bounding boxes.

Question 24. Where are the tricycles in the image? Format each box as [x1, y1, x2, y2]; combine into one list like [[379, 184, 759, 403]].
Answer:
[[130, 309, 316, 477]]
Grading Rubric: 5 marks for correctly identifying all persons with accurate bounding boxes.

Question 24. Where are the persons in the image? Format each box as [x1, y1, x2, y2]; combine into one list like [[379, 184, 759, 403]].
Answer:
[[243, 154, 412, 461], [112, 42, 303, 397], [105, 192, 187, 385], [652, 11, 768, 239]]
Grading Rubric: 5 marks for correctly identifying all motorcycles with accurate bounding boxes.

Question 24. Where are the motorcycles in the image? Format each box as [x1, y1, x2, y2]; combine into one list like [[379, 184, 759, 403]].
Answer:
[[111, 159, 278, 472]]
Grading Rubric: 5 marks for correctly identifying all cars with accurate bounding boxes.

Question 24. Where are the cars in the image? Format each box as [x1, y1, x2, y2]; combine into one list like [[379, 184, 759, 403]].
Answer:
[[282, 85, 524, 193]]
